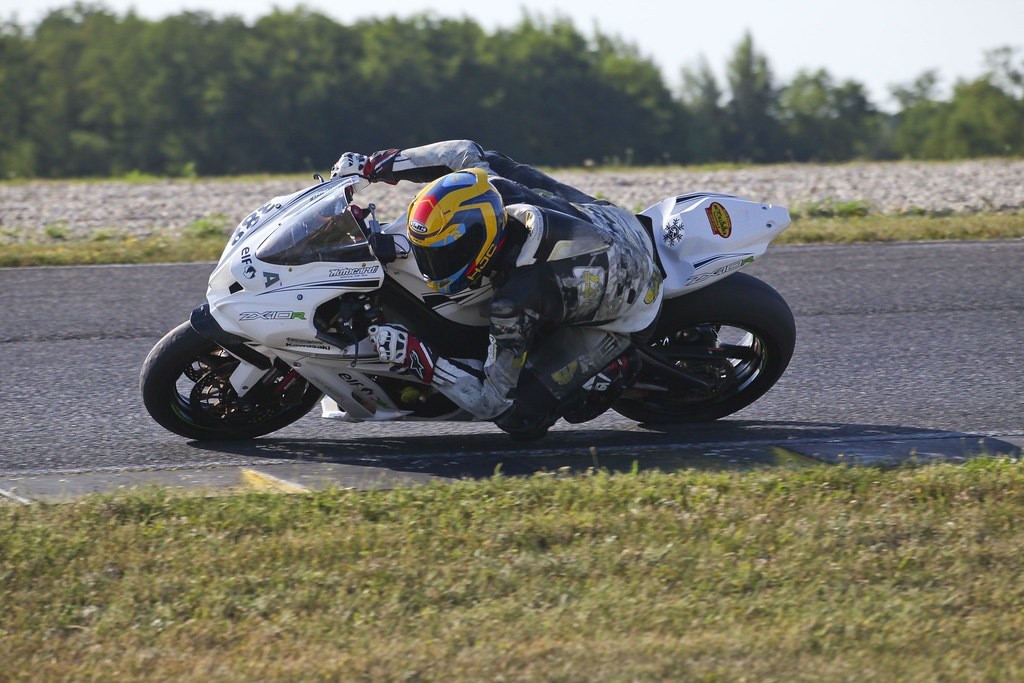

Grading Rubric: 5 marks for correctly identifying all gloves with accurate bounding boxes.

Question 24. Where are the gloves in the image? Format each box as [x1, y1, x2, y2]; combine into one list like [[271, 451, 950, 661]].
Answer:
[[368, 322, 441, 382], [330, 148, 402, 185]]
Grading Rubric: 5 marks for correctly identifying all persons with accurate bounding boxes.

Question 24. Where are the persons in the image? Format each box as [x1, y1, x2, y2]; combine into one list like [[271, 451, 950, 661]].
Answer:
[[330, 140, 664, 433]]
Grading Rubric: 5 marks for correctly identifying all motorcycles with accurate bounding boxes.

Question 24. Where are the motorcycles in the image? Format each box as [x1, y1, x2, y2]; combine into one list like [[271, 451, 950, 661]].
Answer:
[[138, 164, 799, 441]]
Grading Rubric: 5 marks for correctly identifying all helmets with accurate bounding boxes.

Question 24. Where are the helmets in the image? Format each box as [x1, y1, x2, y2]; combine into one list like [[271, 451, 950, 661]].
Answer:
[[407, 168, 509, 296]]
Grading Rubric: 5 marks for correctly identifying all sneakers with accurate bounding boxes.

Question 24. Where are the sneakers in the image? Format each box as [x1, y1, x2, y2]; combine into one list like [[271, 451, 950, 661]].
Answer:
[[563, 352, 642, 424]]
